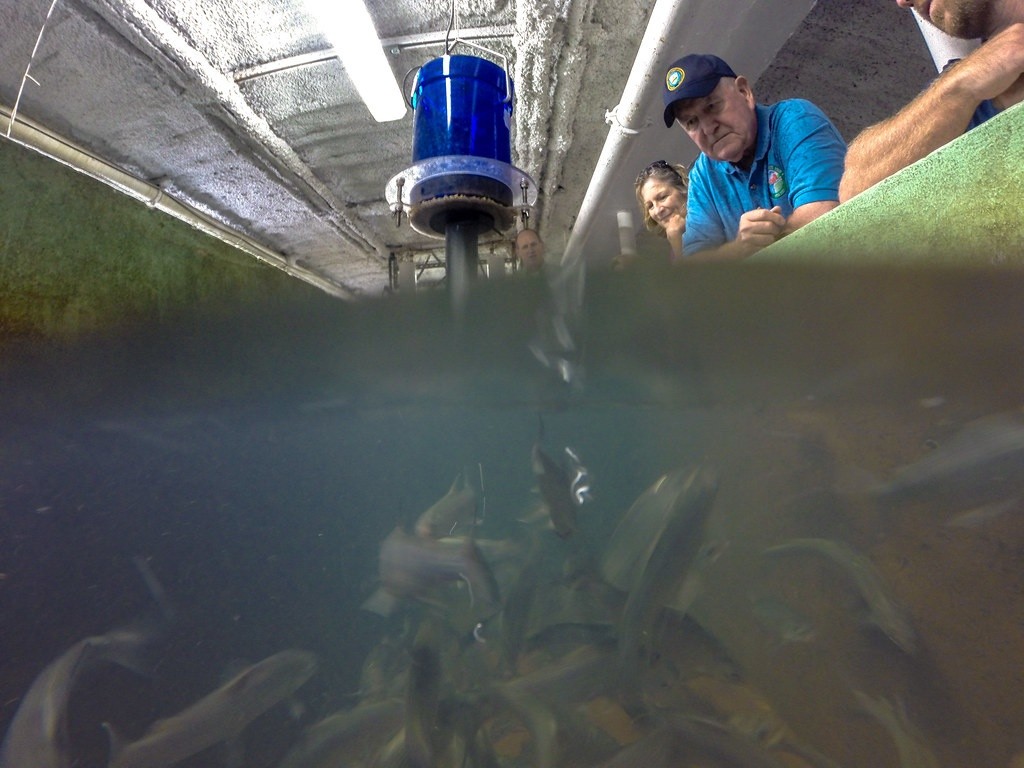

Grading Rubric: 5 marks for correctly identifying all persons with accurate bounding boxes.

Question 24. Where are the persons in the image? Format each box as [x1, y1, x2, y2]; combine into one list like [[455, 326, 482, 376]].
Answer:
[[838, 0, 1022, 205], [515, 228, 547, 269], [636, 161, 690, 260], [661, 54, 849, 268]]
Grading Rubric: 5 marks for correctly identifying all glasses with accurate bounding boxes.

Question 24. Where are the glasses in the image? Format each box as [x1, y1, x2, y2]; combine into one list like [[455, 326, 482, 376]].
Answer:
[[637, 160, 682, 178]]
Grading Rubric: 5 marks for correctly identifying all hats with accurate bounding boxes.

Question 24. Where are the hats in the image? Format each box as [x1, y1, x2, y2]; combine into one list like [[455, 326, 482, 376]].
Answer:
[[662, 54, 738, 128]]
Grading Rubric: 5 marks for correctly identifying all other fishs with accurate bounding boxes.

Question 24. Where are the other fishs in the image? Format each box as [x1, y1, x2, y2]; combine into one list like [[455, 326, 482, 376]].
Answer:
[[0, 414, 1023, 768]]
